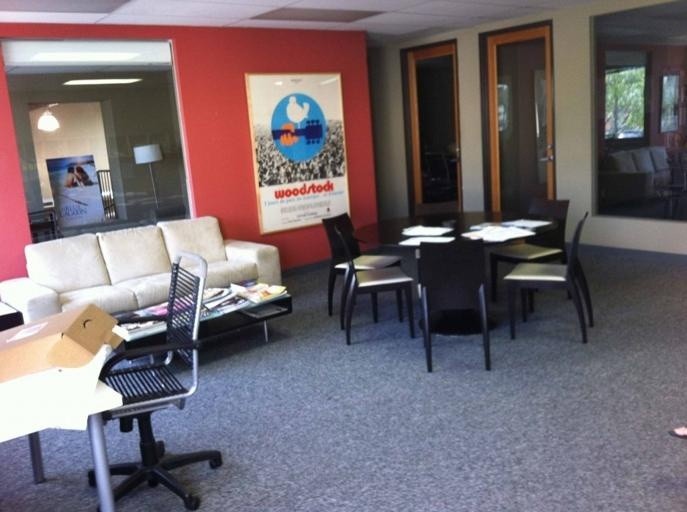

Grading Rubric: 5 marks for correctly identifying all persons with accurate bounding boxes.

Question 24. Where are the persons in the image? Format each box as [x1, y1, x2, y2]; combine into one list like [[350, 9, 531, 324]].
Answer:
[[74, 166, 93, 186], [65, 166, 78, 188]]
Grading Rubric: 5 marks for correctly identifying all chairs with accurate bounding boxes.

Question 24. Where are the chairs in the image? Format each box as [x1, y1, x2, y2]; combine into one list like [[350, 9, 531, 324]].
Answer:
[[85, 252, 222, 512], [322, 198, 595, 374], [25, 209, 56, 244]]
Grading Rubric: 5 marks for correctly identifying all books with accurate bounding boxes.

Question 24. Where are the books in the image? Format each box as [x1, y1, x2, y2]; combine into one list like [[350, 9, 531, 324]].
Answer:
[[113, 282, 288, 333]]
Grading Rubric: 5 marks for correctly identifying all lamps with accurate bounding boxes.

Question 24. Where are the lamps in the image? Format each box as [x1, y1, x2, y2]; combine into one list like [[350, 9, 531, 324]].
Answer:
[[36, 103, 60, 132], [131, 141, 165, 215]]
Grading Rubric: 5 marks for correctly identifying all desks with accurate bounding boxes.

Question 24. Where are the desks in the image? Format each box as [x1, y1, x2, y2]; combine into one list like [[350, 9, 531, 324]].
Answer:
[[1, 377, 124, 510]]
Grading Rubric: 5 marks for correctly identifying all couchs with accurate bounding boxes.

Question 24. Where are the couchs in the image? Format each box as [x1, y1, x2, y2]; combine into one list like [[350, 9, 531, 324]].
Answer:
[[600, 143, 683, 216], [0, 212, 283, 343]]
